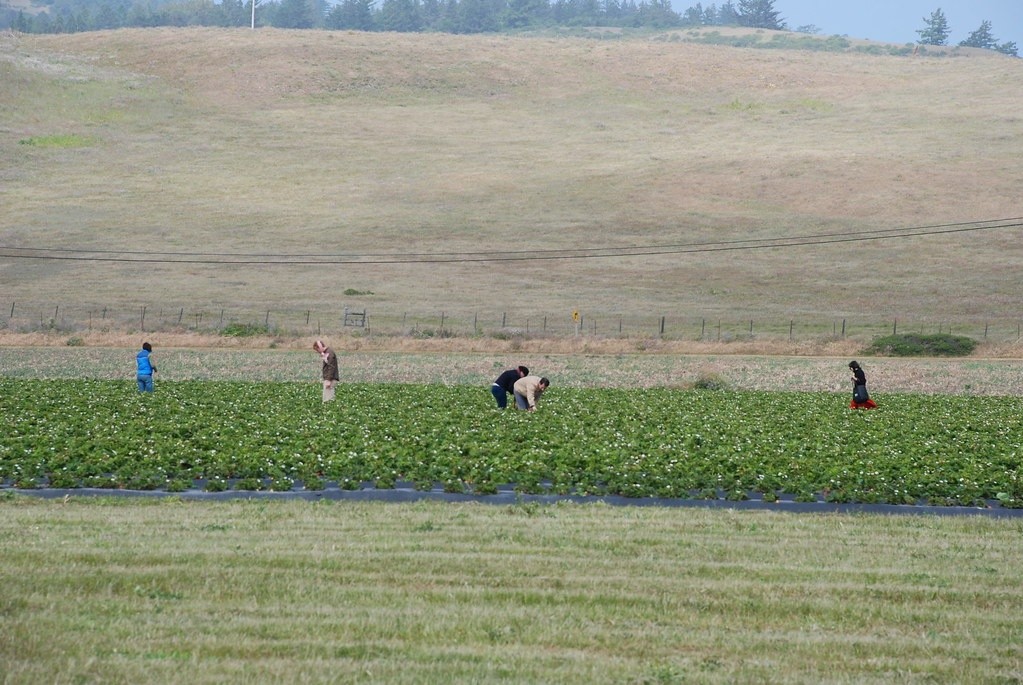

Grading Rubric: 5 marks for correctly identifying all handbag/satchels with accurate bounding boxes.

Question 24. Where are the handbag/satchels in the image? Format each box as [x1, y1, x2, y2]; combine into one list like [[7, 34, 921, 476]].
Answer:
[[851, 399, 877, 409], [853, 378, 868, 404]]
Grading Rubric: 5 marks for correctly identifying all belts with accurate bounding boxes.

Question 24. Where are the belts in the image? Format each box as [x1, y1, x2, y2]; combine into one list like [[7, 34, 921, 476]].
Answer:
[[492, 383, 500, 387]]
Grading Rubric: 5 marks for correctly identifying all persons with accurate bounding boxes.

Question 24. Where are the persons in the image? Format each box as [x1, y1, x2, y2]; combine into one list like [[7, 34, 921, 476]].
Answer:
[[136, 342, 157, 392], [849, 361, 875, 411], [514, 375, 549, 413], [313, 340, 338, 403], [490, 366, 529, 408]]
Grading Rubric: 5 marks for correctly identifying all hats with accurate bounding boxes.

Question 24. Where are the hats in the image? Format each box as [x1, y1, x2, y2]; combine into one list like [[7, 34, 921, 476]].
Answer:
[[518, 366, 529, 377]]
